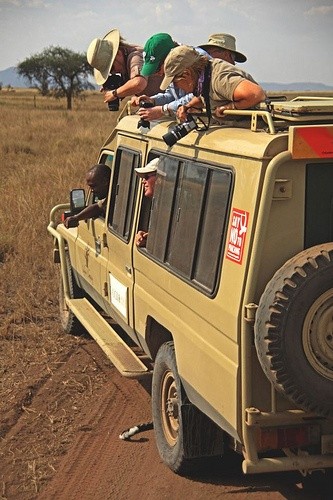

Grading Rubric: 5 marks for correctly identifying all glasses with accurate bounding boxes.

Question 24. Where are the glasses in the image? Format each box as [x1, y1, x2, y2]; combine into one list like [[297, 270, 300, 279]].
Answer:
[[142, 176, 150, 181], [156, 66, 161, 73]]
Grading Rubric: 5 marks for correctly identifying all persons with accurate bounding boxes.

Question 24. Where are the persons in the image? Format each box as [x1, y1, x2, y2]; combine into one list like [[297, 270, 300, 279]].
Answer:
[[199, 33, 247, 65], [130, 33, 212, 121], [87, 29, 165, 104], [134, 158, 159, 247], [63, 164, 111, 230], [160, 44, 270, 121]]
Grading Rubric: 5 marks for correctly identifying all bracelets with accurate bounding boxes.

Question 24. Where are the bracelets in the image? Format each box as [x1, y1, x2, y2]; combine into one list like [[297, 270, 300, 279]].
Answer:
[[232, 103, 236, 110], [113, 89, 118, 97], [164, 104, 169, 115]]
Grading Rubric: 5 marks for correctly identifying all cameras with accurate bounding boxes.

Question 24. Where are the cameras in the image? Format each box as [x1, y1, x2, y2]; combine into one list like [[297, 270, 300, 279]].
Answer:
[[162, 113, 198, 147], [103, 75, 124, 111], [137, 101, 155, 130]]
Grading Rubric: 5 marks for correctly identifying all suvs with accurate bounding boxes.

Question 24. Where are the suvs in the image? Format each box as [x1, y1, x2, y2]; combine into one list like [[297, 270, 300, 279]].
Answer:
[[47, 96, 333, 477]]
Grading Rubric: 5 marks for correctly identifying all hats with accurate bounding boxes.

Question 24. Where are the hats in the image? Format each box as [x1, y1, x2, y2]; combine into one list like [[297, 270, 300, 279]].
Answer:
[[133, 157, 160, 178], [86, 28, 120, 86], [197, 32, 248, 63], [140, 31, 175, 77], [159, 42, 200, 91]]
